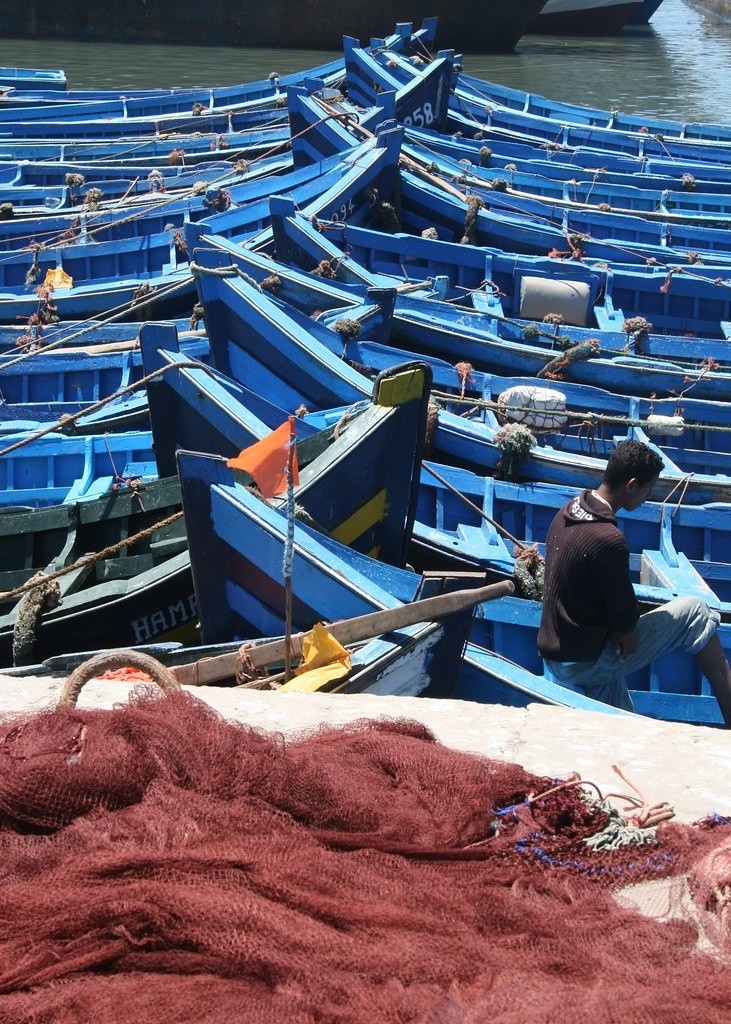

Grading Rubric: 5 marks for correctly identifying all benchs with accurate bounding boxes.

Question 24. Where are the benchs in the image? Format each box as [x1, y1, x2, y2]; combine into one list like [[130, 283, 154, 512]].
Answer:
[[63, 476, 113, 503], [641, 549, 719, 601], [594, 304, 626, 332], [458, 524, 510, 557], [42, 276, 73, 292], [611, 435, 682, 473], [163, 261, 189, 276], [471, 289, 504, 318], [10, 552, 97, 614], [720, 321, 731, 340]]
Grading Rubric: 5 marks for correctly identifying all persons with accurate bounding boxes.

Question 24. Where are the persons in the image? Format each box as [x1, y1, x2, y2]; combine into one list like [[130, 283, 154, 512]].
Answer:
[[535, 438, 731, 730]]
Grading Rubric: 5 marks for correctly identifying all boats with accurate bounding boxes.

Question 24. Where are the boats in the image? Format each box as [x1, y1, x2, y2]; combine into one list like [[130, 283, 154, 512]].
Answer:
[[1, 1, 731, 731]]
